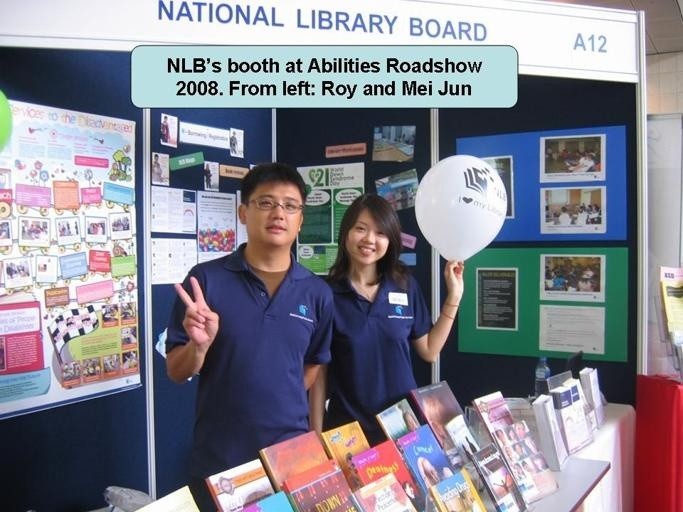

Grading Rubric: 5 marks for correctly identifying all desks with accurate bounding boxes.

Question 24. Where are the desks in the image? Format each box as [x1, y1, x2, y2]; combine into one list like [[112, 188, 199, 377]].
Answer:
[[527, 452, 614, 512]]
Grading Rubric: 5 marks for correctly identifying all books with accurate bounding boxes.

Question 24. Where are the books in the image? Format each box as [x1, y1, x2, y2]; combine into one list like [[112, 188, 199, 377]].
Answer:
[[129, 362, 607, 512], [657, 263, 683, 374]]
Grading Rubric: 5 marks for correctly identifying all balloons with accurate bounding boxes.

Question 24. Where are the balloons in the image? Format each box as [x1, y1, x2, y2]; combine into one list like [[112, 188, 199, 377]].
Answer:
[[413, 153, 508, 265]]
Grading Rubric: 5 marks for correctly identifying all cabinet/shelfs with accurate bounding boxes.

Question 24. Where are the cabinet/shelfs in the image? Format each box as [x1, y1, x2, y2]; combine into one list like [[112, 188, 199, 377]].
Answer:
[[505, 391, 635, 512]]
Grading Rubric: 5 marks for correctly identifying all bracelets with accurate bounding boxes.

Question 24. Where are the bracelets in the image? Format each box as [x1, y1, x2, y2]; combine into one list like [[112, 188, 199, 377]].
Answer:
[[441, 300, 461, 309], [440, 308, 457, 322]]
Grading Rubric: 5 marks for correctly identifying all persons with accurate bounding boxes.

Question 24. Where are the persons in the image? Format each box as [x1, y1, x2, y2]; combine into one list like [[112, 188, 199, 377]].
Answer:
[[163, 162, 336, 499], [0, 214, 131, 281], [153, 110, 245, 189], [55, 301, 139, 381], [306, 191, 473, 447], [542, 138, 601, 292]]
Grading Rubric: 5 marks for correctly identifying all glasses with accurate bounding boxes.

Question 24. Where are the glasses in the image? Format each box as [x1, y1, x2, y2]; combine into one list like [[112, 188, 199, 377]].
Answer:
[[248, 197, 304, 213]]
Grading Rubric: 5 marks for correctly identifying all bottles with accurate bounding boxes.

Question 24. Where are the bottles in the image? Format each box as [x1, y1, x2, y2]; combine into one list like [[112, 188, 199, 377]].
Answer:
[[534, 355, 550, 396]]
